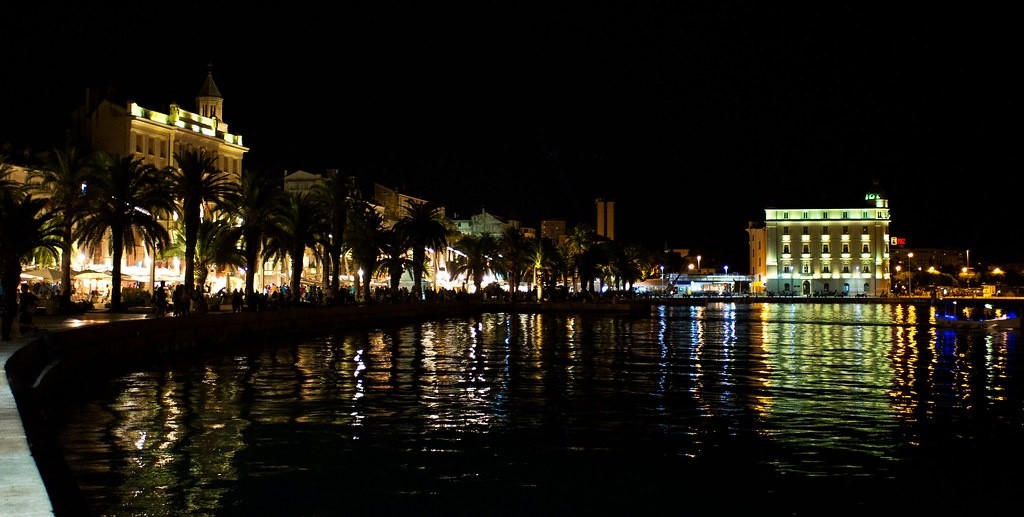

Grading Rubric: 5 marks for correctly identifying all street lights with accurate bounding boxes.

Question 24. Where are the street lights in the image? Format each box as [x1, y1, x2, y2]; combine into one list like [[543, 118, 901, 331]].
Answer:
[[894, 265, 902, 296], [908, 253, 914, 298]]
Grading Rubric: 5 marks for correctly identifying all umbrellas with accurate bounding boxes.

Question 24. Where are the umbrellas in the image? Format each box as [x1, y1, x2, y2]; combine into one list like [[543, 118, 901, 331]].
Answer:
[[21, 263, 249, 292]]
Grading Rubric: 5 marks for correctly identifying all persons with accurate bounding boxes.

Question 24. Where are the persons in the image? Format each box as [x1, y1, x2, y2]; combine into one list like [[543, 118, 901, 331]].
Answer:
[[17, 285, 674, 319], [857, 266, 1004, 301]]
